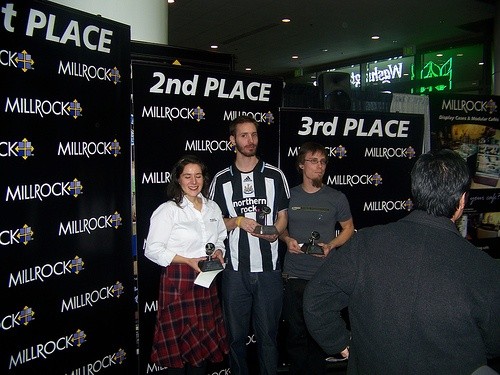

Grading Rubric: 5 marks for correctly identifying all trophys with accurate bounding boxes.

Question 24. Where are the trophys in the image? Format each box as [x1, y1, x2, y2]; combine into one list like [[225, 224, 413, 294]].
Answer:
[[300, 231, 325, 255], [198, 243, 224, 272], [253, 205, 280, 235]]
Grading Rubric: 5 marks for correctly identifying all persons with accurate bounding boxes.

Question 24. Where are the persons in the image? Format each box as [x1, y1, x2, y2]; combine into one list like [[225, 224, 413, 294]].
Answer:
[[303, 148, 500, 375], [150, 156, 232, 375], [283, 144, 356, 375], [206, 116, 291, 375]]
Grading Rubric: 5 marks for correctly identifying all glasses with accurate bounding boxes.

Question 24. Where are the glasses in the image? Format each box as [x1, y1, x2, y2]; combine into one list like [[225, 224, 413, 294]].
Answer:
[[305, 158, 329, 165]]
